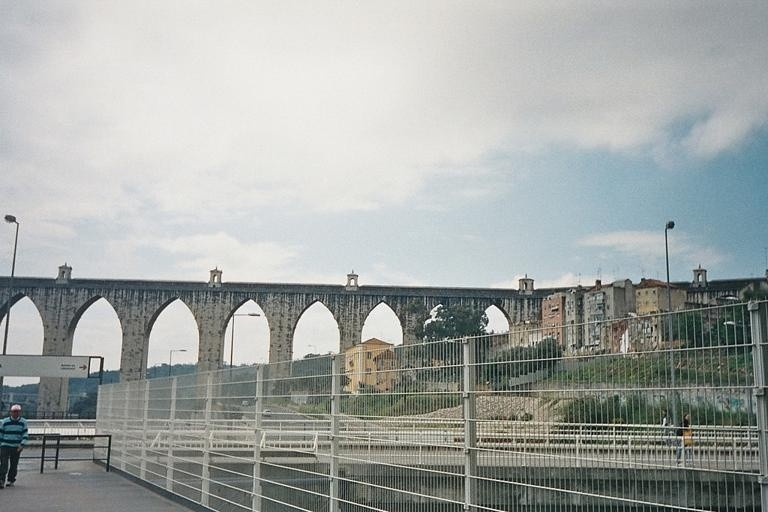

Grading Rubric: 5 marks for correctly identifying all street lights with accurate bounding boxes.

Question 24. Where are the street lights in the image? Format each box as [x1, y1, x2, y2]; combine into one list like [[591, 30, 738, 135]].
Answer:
[[401, 364, 407, 412], [166, 349, 187, 440], [227, 313, 261, 440], [153, 361, 167, 377], [723, 320, 752, 329], [0, 215, 19, 420], [665, 219, 682, 465]]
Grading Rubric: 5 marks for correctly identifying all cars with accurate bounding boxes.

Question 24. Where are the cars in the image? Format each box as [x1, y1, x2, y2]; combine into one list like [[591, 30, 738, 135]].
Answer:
[[567, 343, 611, 363], [327, 412, 352, 436], [241, 400, 250, 407], [262, 408, 272, 417], [223, 405, 243, 420]]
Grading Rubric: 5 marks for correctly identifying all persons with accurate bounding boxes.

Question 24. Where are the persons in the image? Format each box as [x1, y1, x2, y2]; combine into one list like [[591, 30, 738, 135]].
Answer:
[[677, 413, 690, 463], [0, 404, 29, 489], [662, 407, 672, 425]]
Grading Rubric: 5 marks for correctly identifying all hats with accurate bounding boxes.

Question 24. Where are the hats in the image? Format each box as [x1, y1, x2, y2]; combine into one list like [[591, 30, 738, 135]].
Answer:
[[11, 405, 21, 412]]
